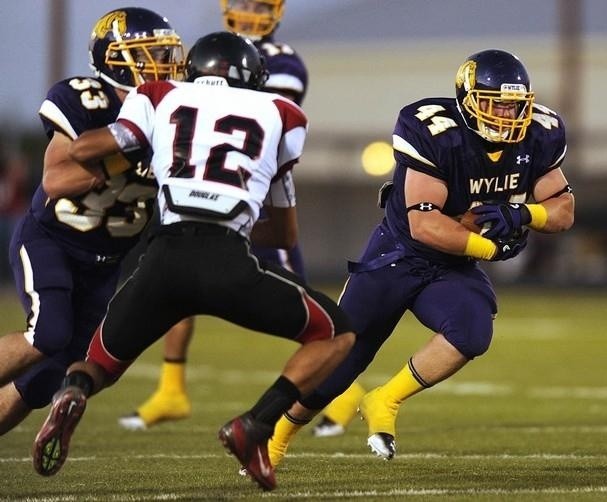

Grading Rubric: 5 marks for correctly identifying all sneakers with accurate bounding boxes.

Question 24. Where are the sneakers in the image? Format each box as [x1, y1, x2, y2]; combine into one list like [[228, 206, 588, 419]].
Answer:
[[357, 385, 400, 460], [309, 380, 366, 436], [117, 388, 192, 432], [218, 409, 290, 490], [31, 385, 87, 476]]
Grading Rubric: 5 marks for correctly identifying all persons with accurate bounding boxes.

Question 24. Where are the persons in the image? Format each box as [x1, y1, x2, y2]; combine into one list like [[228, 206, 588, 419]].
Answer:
[[1, 5, 185, 439], [32, 29, 358, 492], [259, 46, 578, 470], [115, 0, 366, 438]]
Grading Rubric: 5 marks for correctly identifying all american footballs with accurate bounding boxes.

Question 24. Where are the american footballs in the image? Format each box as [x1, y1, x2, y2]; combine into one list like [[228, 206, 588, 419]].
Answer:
[[460, 211, 495, 235]]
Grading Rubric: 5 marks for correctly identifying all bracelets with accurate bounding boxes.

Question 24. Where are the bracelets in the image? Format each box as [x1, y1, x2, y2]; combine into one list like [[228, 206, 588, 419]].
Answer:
[[100, 152, 133, 181], [523, 202, 549, 232], [463, 231, 497, 262]]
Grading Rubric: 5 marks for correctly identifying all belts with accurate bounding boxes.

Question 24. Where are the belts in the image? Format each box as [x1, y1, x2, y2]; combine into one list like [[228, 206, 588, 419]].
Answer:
[[56, 238, 119, 268]]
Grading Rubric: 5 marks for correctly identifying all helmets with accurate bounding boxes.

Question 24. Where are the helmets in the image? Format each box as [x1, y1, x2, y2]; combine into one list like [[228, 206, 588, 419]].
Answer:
[[87, 7, 186, 93], [185, 31, 269, 90], [455, 49, 535, 144], [219, 0, 285, 37]]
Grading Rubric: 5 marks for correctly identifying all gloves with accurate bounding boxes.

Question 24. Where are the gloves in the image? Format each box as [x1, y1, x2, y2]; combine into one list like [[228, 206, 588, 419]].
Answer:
[[491, 227, 529, 262], [470, 200, 531, 241]]
